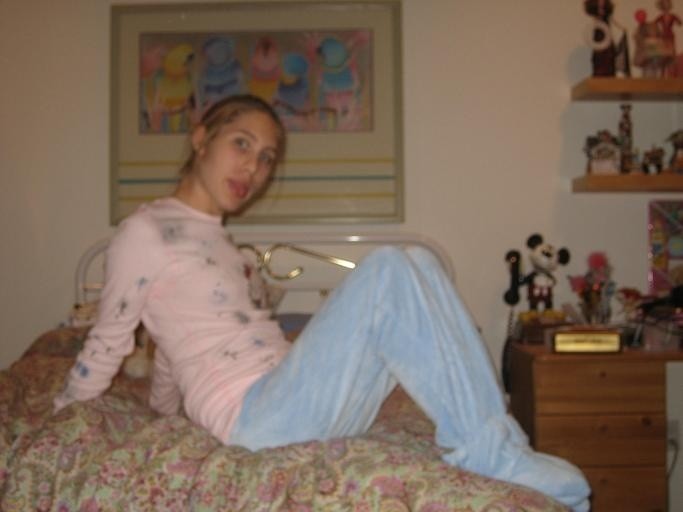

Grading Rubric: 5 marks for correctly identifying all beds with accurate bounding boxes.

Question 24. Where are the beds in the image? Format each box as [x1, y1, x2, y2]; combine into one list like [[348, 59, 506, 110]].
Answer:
[[0, 230, 569, 511]]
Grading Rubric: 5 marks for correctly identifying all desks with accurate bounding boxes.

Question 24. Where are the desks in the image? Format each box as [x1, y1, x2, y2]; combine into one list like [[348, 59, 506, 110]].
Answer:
[[505, 340, 683, 511]]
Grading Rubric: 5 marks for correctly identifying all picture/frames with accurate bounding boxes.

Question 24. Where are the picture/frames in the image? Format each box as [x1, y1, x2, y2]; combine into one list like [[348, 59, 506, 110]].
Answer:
[[108, 0, 406, 226]]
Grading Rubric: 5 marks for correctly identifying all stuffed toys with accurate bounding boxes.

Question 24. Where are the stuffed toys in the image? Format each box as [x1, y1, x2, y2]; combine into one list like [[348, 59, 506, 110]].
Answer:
[[497, 233, 574, 327]]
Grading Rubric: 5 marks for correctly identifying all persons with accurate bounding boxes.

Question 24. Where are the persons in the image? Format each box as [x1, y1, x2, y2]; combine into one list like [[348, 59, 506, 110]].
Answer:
[[574, 1, 682, 176], [43, 89, 599, 512]]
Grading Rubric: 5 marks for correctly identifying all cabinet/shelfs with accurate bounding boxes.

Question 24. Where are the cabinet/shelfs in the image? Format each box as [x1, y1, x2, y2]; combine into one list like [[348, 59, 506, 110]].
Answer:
[[569, 76, 682, 190]]
